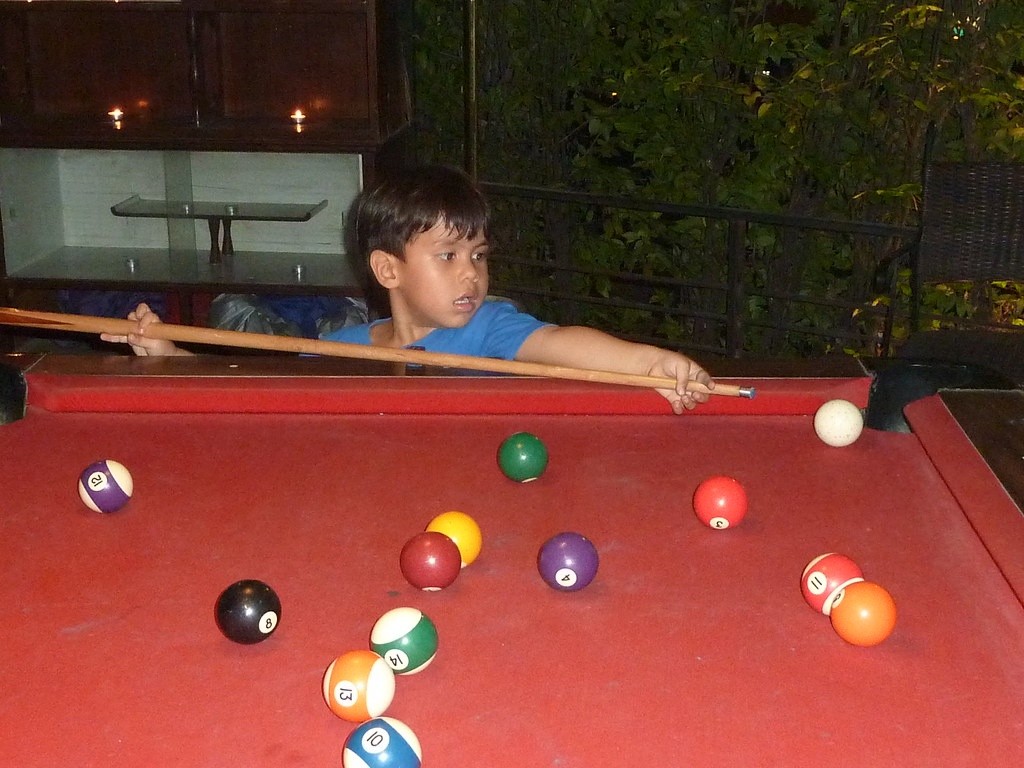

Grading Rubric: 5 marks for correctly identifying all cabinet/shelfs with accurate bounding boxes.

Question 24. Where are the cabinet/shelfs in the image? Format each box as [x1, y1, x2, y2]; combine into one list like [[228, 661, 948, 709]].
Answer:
[[1, 121, 409, 293]]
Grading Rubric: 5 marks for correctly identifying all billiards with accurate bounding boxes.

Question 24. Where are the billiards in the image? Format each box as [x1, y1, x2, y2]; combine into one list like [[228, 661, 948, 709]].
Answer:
[[78, 399, 898, 768]]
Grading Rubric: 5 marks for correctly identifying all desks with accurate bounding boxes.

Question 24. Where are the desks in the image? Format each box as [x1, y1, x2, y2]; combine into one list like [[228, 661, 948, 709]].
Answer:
[[0, 341, 1024, 765], [110, 194, 328, 264]]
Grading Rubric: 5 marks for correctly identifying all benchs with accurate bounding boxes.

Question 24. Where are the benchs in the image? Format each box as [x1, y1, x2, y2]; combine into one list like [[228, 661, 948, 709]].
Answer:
[[874, 124, 1023, 383]]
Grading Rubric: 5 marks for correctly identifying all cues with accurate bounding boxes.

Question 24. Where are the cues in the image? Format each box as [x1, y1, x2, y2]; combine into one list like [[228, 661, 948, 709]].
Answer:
[[0, 306, 756, 399]]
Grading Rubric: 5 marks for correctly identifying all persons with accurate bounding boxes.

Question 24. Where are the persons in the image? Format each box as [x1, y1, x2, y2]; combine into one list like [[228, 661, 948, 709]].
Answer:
[[99, 162, 717, 416]]
